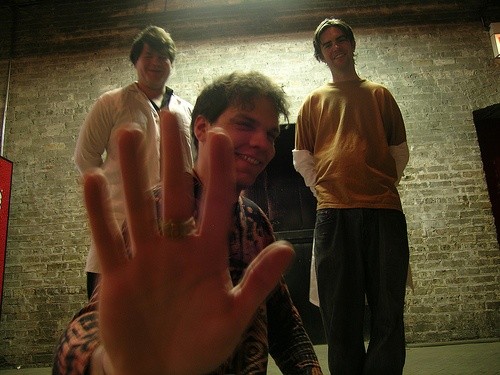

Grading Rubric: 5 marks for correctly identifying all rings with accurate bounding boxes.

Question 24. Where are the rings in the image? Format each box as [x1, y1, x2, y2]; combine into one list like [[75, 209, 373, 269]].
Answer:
[[160, 215, 197, 238]]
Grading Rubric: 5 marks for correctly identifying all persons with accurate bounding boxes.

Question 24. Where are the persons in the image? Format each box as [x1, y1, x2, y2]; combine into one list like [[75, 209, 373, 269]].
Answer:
[[73, 26, 198, 304], [292, 18, 412, 374], [51, 70, 322, 374]]
